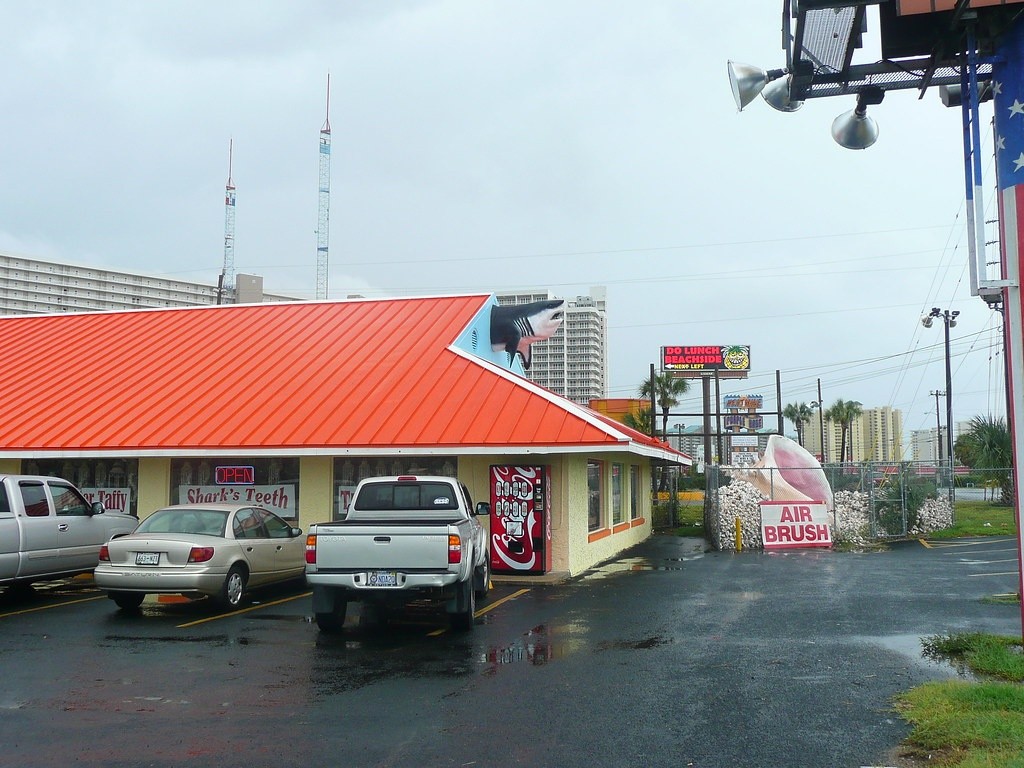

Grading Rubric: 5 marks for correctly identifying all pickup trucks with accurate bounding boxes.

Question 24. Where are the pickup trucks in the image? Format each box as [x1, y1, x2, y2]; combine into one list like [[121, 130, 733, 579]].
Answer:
[[0, 474, 140, 585], [304, 476, 492, 636]]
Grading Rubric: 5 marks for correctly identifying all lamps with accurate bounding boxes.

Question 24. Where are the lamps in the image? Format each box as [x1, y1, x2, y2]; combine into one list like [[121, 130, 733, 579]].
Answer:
[[728, 59, 814, 112], [831, 85, 885, 150], [761, 73, 805, 112], [939, 80, 990, 107]]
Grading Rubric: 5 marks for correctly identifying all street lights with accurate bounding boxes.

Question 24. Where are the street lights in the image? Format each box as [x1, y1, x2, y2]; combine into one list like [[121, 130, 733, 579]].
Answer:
[[811, 403, 824, 466], [674, 424, 686, 477], [846, 446, 849, 463], [922, 306, 960, 502], [924, 412, 941, 468], [890, 439, 896, 465]]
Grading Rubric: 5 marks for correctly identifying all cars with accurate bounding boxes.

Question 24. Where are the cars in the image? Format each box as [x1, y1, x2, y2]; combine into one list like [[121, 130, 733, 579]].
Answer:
[[651, 479, 660, 491], [93, 503, 307, 609], [866, 472, 892, 487]]
